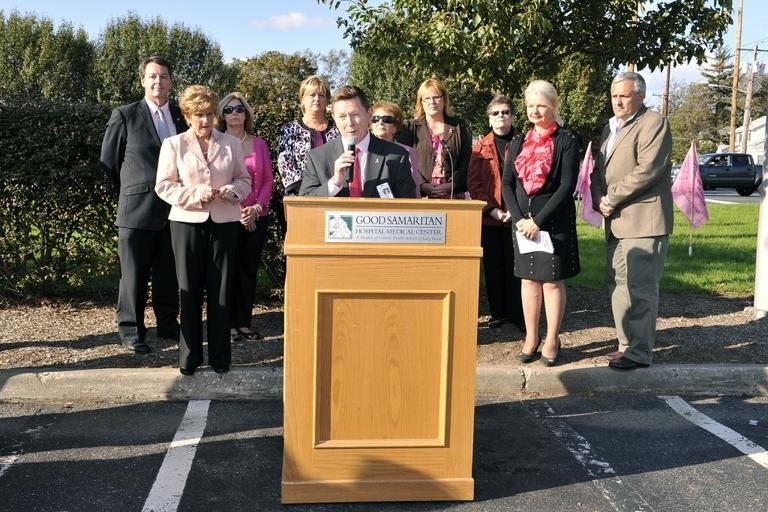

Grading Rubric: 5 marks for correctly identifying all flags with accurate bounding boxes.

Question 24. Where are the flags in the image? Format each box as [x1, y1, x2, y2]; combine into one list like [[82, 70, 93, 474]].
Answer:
[[575, 141, 604, 229], [670, 140, 710, 231]]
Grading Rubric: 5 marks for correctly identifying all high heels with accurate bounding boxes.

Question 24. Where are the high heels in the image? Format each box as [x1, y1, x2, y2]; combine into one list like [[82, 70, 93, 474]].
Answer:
[[539, 337, 562, 367], [516, 337, 542, 364]]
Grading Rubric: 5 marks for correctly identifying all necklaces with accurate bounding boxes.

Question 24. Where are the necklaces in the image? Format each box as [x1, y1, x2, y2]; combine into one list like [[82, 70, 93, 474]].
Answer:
[[201, 151, 209, 155], [223, 129, 247, 144]]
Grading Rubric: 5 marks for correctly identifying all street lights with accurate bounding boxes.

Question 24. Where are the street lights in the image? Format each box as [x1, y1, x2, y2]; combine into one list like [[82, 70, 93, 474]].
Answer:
[[705, 45, 758, 154]]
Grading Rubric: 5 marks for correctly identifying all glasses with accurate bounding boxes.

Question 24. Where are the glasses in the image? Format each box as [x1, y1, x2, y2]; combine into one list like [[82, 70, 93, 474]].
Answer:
[[223, 104, 246, 114], [370, 115, 398, 124], [490, 109, 511, 116], [420, 94, 444, 103]]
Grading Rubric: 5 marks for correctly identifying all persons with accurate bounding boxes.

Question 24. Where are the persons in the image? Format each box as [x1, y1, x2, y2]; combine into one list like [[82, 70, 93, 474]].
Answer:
[[714, 156, 727, 166], [401, 79, 471, 200], [466, 95, 527, 332], [215, 91, 274, 342], [100, 56, 189, 356], [501, 78, 580, 367], [297, 86, 417, 198], [276, 76, 341, 197], [155, 84, 252, 377], [590, 71, 674, 371], [368, 102, 422, 199]]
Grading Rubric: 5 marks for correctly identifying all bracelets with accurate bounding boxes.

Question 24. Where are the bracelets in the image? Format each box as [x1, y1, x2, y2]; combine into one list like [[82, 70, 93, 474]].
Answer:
[[608, 203, 615, 209]]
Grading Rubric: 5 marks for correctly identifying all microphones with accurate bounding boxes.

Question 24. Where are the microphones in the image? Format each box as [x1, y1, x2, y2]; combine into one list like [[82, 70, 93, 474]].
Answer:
[[440, 139, 454, 199], [346, 136, 357, 183]]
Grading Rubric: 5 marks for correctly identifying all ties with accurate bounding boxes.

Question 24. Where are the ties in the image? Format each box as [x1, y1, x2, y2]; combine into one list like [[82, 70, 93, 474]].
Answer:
[[155, 106, 172, 145], [347, 145, 364, 198]]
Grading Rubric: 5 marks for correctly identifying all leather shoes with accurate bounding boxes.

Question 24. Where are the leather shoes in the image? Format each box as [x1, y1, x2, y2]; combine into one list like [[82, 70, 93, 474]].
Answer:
[[607, 350, 651, 372], [211, 364, 231, 374], [179, 366, 198, 377], [230, 325, 262, 344], [120, 339, 153, 356]]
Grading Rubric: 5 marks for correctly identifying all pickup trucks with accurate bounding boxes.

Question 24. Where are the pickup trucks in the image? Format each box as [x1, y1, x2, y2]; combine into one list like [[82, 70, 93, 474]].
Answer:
[[671, 152, 762, 197]]
